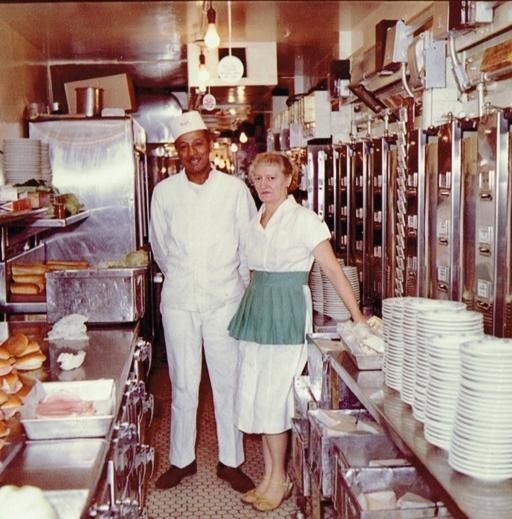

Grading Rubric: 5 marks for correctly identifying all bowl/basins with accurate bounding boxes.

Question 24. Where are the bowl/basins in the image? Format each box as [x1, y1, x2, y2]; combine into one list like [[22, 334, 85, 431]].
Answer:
[[19, 378, 117, 441]]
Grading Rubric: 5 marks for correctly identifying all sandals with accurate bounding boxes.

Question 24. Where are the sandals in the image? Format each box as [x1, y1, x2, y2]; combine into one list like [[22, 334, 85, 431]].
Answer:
[[242, 477, 272, 503], [252, 474, 294, 511]]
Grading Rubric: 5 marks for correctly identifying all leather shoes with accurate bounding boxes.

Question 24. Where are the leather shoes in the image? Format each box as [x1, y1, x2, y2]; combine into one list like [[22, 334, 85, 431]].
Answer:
[[219, 463, 256, 492], [155, 461, 197, 487]]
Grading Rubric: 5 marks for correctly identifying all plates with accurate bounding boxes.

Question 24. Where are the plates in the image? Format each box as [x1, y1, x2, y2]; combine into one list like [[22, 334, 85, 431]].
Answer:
[[423, 332, 499, 454], [320, 266, 360, 320], [411, 308, 484, 424], [336, 328, 383, 371], [400, 299, 467, 407], [2, 138, 54, 186], [447, 338, 511, 485], [382, 297, 433, 394], [310, 259, 345, 314]]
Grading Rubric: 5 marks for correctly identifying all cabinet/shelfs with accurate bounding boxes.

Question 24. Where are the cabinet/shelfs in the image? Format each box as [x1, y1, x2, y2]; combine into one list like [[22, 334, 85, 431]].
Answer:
[[305, 334, 512, 519], [0, 322, 145, 519]]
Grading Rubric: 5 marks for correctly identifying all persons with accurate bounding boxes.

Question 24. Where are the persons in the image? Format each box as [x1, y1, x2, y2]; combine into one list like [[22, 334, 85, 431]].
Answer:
[[147, 111, 258, 493], [225, 151, 371, 510]]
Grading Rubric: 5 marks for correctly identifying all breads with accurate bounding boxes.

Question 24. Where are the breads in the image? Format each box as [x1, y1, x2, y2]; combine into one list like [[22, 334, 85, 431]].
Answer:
[[12, 276, 44, 287], [8, 282, 39, 296], [1, 333, 27, 356], [12, 352, 46, 370], [19, 341, 41, 355], [47, 261, 87, 271], [0, 359, 34, 461], [13, 263, 50, 275]]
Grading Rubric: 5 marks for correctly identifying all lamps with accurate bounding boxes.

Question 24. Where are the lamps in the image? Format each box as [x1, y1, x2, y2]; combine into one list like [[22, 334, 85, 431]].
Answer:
[[196, 40, 212, 91], [203, 0, 221, 50], [216, 0, 246, 85]]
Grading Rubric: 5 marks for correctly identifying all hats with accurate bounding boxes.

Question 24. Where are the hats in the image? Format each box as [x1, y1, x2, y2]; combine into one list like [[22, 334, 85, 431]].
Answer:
[[172, 111, 208, 142]]
